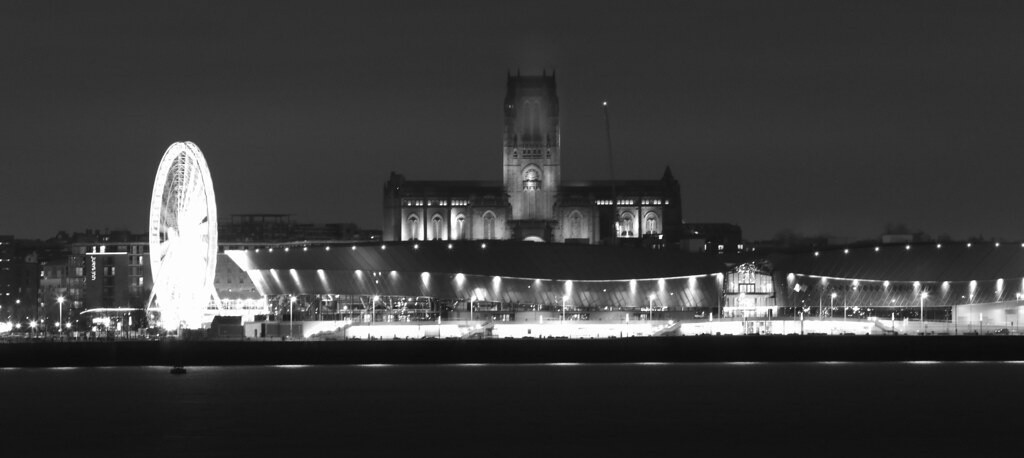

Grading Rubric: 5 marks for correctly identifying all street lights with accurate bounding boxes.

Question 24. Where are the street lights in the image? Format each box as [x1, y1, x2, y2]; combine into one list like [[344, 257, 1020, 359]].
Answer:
[[562, 295, 569, 319], [831, 292, 837, 317], [58, 297, 64, 338], [471, 296, 476, 319], [650, 294, 656, 319], [290, 297, 297, 339], [921, 291, 928, 333], [373, 296, 379, 337]]
